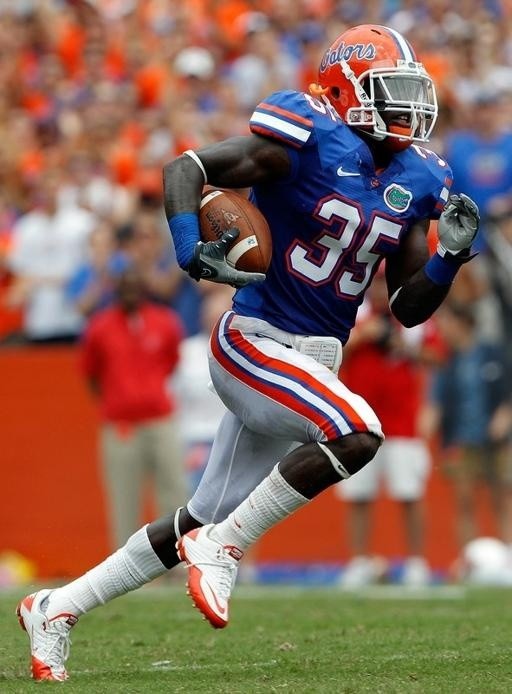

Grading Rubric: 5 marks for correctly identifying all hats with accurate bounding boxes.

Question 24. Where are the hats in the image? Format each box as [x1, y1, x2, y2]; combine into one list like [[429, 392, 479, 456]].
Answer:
[[171, 45, 216, 81]]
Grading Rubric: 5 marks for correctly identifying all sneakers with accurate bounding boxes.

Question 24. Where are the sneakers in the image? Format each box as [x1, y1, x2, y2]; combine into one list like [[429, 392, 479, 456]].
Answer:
[[333, 551, 435, 592], [14, 585, 81, 685], [171, 520, 241, 629]]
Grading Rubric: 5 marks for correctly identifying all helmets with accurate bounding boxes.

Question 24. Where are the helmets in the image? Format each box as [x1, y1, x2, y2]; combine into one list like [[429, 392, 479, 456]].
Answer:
[[315, 18, 432, 152]]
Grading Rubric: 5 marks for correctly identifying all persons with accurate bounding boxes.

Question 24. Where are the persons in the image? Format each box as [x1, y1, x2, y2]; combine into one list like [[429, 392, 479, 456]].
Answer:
[[164, 293, 229, 489], [420, 311, 508, 589], [15, 19, 485, 682], [338, 254, 450, 595], [1, 0, 512, 340], [81, 268, 188, 550]]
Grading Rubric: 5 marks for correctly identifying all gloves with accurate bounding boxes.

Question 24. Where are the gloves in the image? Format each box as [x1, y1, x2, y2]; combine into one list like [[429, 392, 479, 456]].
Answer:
[[185, 226, 267, 291], [433, 191, 482, 261]]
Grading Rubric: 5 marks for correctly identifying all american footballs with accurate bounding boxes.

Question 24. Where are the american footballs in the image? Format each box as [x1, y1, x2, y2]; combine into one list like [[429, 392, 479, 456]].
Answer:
[[199, 189, 272, 273]]
